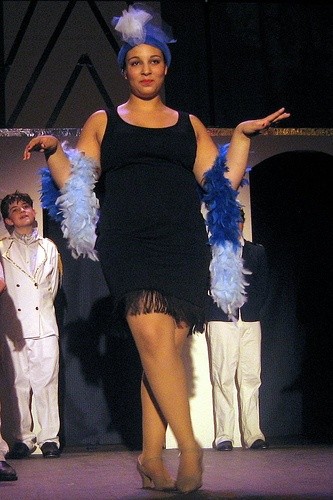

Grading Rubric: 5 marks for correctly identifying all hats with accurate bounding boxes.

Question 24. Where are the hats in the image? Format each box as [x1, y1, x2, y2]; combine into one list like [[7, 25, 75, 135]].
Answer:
[[111, 3, 178, 67]]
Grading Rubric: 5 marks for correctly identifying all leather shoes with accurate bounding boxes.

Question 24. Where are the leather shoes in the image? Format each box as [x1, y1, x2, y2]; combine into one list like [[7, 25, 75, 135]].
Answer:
[[250, 439, 267, 449], [12, 442, 29, 459], [217, 441, 232, 451], [41, 442, 59, 458], [0, 460, 18, 481]]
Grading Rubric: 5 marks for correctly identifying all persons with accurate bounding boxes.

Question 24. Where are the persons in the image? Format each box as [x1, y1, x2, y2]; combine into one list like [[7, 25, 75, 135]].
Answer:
[[203, 208, 269, 451], [23, 5, 290, 496], [0, 190, 61, 481]]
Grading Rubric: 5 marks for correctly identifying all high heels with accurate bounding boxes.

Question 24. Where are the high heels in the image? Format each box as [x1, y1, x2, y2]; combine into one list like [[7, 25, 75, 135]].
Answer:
[[137, 454, 177, 490], [176, 448, 204, 494]]
